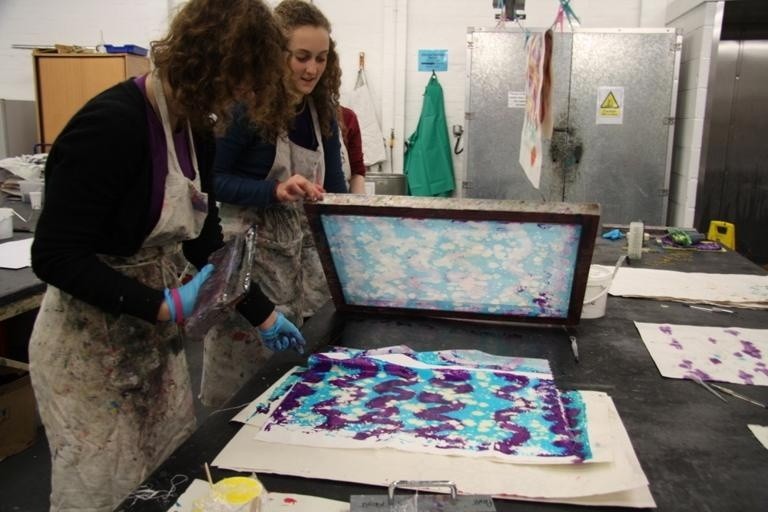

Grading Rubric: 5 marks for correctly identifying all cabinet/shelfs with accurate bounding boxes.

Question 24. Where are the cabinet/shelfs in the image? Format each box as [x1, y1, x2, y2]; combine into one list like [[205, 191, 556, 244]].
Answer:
[[31, 48, 151, 158]]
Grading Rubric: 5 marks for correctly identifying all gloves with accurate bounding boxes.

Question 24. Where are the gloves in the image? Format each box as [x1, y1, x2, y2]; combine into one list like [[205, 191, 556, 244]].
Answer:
[[164, 262, 217, 325], [254, 312, 308, 357]]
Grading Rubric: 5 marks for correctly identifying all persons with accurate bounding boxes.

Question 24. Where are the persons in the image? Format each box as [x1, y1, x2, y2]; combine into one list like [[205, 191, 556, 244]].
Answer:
[[338, 104, 367, 198], [28, 1, 307, 512], [199, 1, 348, 410]]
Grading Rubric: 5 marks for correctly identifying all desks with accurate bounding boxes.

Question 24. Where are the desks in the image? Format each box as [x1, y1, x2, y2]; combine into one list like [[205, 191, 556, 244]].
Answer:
[[2, 153, 80, 373], [113, 223, 768, 512]]
